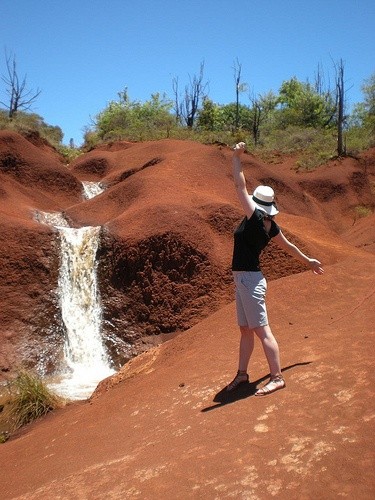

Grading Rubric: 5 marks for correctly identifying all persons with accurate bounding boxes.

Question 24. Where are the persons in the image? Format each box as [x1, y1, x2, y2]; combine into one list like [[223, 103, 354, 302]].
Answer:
[[225, 141, 322, 396]]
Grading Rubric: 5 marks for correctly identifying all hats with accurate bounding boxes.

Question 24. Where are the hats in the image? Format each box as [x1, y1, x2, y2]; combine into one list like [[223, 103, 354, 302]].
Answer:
[[248, 185, 279, 216]]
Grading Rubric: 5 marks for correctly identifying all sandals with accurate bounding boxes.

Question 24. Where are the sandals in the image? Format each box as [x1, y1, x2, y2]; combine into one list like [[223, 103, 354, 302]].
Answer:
[[254, 374, 285, 396], [223, 370, 249, 393]]
